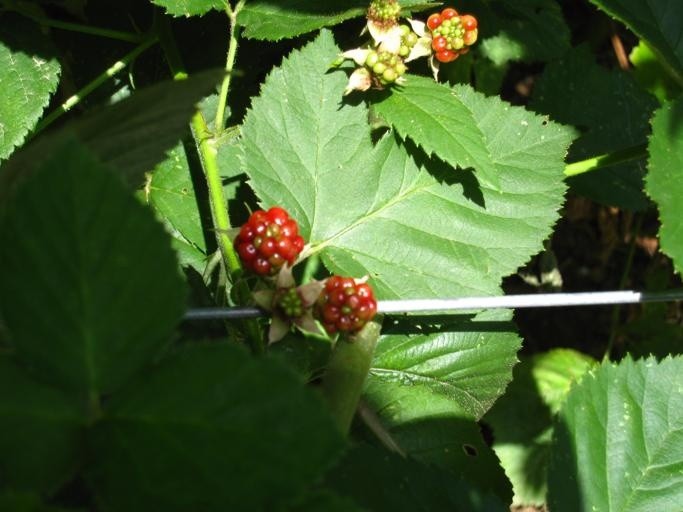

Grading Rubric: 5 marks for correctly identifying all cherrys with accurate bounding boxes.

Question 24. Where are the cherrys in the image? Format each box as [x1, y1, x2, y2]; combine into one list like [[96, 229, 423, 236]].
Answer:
[[366, 0, 477, 85], [233, 207, 376, 332]]
